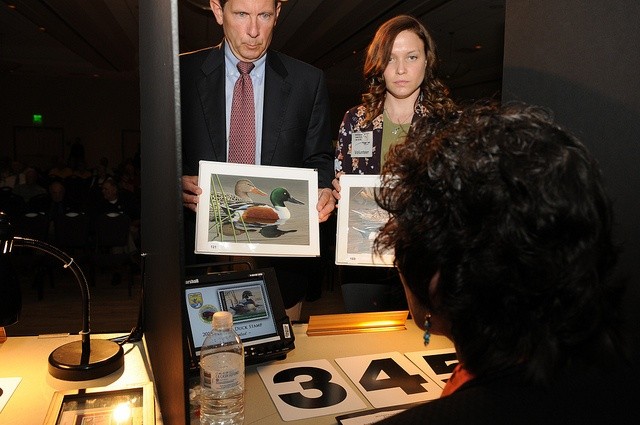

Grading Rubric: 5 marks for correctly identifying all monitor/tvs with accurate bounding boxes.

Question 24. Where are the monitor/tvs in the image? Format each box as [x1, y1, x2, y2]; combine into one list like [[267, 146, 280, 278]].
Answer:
[[183, 267, 295, 378]]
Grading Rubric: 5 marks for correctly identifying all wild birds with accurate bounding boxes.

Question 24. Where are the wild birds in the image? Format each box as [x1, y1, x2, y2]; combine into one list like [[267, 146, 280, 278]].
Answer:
[[230, 290, 263, 315], [234, 301, 256, 315]]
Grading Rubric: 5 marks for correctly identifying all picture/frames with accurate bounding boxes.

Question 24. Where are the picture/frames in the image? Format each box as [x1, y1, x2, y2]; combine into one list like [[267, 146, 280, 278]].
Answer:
[[334, 174, 401, 267], [194, 160, 323, 258]]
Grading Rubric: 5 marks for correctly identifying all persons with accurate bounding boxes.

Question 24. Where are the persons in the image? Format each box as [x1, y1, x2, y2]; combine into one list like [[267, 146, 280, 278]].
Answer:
[[331, 15, 460, 313], [368, 97, 639, 425], [182, 1, 336, 325], [0, 155, 140, 328]]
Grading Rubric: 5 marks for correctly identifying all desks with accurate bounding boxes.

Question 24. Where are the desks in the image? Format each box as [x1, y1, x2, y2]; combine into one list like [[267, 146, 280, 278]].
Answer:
[[191, 318, 462, 425], [0, 331, 168, 425]]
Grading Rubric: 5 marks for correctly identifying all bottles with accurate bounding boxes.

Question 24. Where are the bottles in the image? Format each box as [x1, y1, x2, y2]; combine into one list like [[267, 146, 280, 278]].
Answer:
[[199, 312, 246, 425]]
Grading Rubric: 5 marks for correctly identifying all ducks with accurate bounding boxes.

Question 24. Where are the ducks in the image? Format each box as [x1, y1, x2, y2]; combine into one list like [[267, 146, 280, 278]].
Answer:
[[221, 186, 308, 228], [210, 178, 269, 220]]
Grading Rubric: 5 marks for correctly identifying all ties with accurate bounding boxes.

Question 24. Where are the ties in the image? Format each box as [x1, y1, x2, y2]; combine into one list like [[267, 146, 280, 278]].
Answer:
[[227, 62, 256, 165]]
